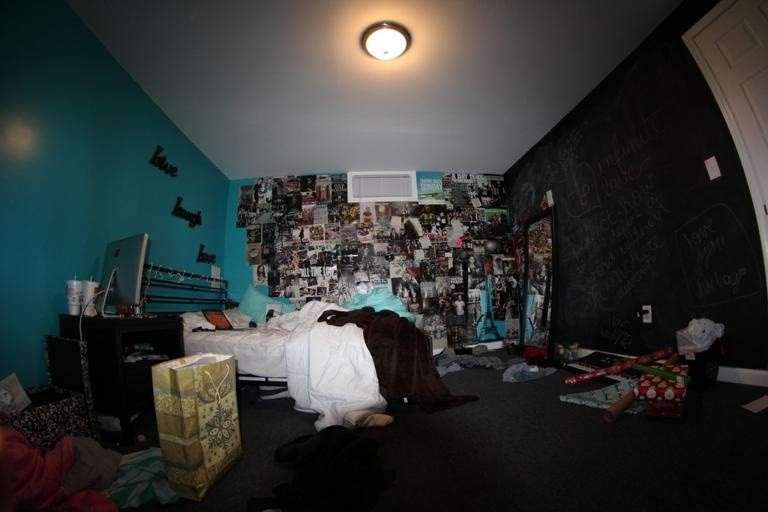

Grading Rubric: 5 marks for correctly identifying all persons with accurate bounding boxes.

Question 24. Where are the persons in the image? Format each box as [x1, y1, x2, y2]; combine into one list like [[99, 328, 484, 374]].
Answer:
[[235, 174, 521, 350]]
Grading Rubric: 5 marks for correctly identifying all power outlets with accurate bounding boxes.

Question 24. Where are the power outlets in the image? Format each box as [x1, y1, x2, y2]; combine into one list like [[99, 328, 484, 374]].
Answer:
[[642, 305, 652, 324]]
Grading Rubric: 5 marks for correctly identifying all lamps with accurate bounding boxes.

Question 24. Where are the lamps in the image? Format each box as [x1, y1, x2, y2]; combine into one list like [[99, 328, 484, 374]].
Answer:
[[361, 23, 412, 61]]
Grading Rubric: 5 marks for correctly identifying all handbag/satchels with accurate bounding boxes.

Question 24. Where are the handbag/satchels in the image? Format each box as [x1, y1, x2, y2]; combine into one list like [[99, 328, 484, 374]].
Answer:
[[151, 351, 243, 502]]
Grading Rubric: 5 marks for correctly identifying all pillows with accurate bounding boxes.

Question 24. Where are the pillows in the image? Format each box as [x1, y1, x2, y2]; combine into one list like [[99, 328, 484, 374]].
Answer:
[[178, 307, 256, 333]]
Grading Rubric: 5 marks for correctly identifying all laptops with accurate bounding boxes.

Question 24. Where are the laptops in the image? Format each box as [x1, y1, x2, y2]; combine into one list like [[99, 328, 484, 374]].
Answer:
[[201, 308, 247, 332]]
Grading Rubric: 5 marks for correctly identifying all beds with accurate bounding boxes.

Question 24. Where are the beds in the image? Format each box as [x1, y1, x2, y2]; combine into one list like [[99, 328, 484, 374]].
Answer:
[[135, 262, 427, 405]]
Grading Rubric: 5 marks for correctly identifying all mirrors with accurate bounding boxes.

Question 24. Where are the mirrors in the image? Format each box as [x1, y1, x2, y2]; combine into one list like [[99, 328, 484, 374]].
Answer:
[[516, 205, 559, 369]]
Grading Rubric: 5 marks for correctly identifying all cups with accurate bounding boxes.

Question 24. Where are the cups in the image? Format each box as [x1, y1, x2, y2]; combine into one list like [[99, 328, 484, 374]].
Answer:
[[66, 279, 84, 316], [82, 280, 100, 317]]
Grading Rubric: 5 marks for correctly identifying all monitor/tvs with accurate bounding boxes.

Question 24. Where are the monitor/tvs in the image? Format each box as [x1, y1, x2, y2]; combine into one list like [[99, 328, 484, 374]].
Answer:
[[99, 232, 150, 318]]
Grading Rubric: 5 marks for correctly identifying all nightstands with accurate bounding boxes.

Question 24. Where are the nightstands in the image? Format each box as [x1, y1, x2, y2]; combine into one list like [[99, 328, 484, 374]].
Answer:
[[57, 313, 185, 456]]
